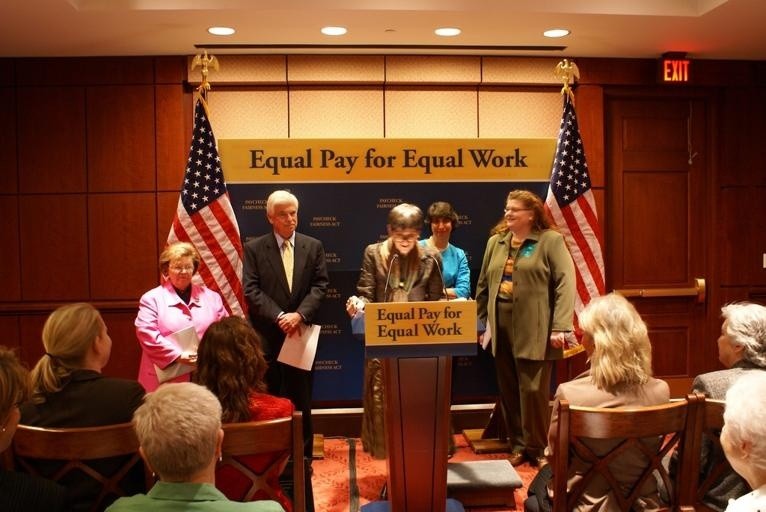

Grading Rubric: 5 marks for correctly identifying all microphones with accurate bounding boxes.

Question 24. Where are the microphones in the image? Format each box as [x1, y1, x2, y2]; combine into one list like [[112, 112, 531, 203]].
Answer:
[[421, 254, 449, 301], [384, 254, 398, 302]]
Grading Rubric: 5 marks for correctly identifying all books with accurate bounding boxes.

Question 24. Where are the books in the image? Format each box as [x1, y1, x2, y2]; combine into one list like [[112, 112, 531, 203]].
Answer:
[[276, 323, 322, 372], [152, 325, 201, 384]]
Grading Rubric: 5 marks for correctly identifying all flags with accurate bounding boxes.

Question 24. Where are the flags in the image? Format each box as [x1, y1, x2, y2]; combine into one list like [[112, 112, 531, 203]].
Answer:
[[543, 88, 608, 361], [161, 90, 248, 319]]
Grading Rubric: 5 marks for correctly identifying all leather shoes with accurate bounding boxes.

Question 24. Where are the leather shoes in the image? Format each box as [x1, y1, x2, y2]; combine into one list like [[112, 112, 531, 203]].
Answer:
[[535, 455, 550, 471], [507, 451, 527, 466]]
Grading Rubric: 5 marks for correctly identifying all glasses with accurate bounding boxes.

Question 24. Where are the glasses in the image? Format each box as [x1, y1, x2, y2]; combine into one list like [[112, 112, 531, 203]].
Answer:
[[503, 207, 535, 213], [391, 229, 420, 243]]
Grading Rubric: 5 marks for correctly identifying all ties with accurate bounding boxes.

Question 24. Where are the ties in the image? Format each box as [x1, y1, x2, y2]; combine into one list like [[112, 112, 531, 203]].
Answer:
[[282, 239, 295, 294]]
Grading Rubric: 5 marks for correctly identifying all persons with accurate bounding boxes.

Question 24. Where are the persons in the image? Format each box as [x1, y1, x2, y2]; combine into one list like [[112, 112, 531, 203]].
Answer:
[[20, 302, 148, 493], [521, 292, 669, 511], [101, 381, 284, 511], [195, 315, 298, 510], [662, 300, 765, 510], [135, 243, 228, 392], [474, 188, 578, 469], [243, 190, 333, 474], [1, 347, 75, 512], [420, 200, 472, 460], [717, 367, 764, 511], [344, 203, 444, 499]]
